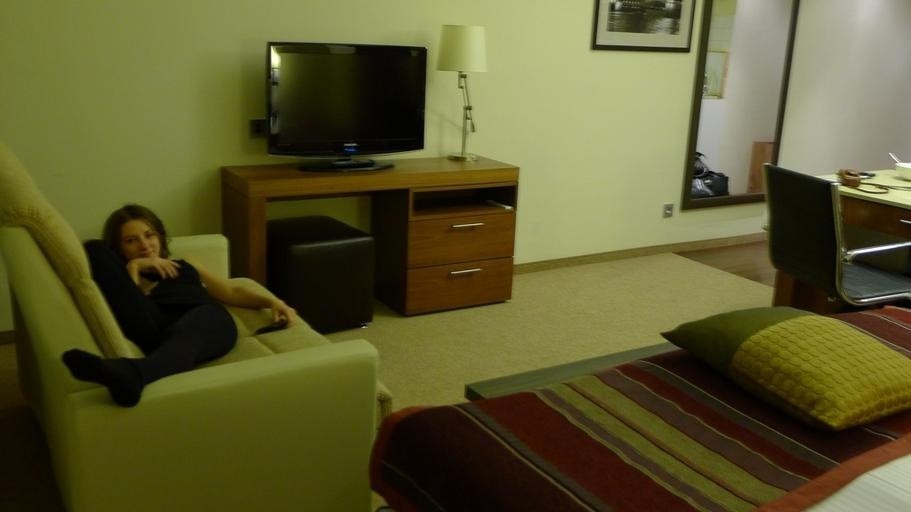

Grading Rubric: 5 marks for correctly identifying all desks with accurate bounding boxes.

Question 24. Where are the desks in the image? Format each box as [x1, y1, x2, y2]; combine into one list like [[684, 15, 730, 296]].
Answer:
[[463, 341, 681, 399]]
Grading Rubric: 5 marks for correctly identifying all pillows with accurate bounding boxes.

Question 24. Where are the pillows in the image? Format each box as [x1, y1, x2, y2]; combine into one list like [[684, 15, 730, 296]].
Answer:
[[658, 303, 911, 432]]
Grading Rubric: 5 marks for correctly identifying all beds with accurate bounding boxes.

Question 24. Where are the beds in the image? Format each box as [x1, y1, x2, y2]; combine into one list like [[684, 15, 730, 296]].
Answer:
[[367, 298, 909, 511]]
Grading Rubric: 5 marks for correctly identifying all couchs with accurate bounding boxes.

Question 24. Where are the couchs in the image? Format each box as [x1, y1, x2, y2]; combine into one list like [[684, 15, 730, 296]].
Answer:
[[1, 220, 392, 510]]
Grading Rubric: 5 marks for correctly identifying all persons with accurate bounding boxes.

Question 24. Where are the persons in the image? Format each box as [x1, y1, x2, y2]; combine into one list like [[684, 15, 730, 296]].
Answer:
[[60, 204, 297, 409]]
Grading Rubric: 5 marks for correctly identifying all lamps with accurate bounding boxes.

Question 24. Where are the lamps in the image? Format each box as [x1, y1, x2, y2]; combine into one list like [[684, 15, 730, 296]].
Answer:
[[433, 25, 491, 164]]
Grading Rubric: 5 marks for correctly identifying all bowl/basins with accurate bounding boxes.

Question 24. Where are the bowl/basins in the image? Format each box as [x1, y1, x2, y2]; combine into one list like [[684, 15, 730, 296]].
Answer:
[[895, 162, 911, 182]]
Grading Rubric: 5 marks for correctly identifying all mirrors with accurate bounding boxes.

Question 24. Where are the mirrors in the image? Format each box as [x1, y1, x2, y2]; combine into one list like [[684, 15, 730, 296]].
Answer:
[[681, 0, 805, 211]]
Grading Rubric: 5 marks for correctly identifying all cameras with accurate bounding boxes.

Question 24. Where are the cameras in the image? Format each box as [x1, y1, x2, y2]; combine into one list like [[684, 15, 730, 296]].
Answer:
[[840, 169, 861, 187]]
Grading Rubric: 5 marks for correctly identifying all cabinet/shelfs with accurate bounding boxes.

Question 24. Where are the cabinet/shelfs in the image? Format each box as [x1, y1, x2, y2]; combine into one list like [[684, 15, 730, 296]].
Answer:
[[219, 152, 519, 317]]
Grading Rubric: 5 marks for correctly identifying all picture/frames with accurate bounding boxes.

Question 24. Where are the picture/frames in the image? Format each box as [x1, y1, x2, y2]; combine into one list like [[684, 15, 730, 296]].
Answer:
[[588, 0, 696, 54]]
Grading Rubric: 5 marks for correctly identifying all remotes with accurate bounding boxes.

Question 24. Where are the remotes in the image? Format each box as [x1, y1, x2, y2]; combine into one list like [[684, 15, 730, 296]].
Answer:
[[257, 318, 287, 335]]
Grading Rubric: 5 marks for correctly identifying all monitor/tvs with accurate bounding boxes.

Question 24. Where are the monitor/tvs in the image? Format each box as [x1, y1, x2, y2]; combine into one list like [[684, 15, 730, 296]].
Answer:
[[267, 42, 426, 171]]
